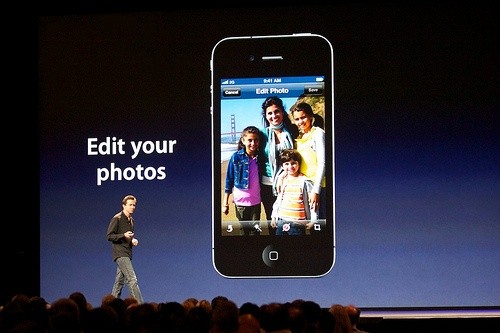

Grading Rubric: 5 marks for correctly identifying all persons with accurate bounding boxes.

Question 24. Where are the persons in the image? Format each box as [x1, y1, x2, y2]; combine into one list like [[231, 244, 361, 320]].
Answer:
[[270, 149, 315, 235], [290, 101, 326, 219], [223, 125, 263, 236], [0, 292, 371, 332], [105, 194, 144, 304], [260, 94, 299, 235]]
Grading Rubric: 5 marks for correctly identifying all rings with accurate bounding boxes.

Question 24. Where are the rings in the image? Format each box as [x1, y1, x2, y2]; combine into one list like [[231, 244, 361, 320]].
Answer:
[[317, 202, 320, 206]]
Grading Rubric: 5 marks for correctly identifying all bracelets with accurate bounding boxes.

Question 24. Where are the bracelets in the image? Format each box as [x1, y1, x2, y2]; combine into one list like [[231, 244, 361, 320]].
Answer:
[[225, 203, 229, 207]]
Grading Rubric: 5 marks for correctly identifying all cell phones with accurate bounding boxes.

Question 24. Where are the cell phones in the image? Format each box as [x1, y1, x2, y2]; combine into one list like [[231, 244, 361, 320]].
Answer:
[[209, 33, 337, 280]]
